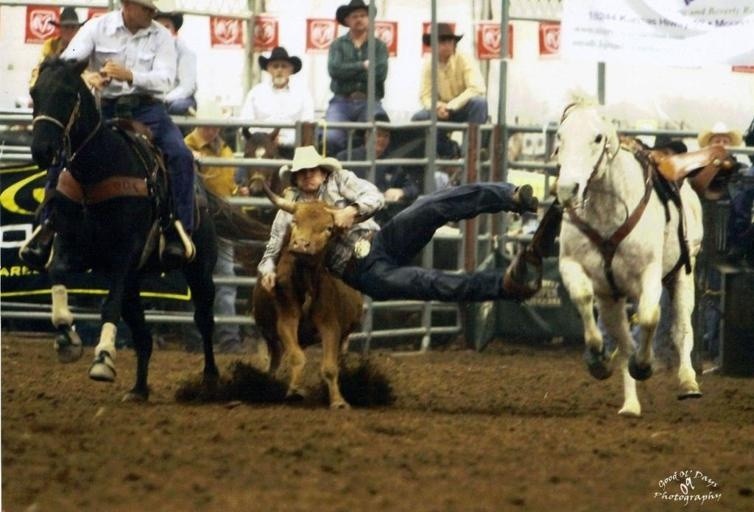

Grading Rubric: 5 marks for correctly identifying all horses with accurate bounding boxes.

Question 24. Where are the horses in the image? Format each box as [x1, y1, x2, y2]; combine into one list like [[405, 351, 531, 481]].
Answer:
[[31, 57, 271, 400], [551, 95, 703, 418]]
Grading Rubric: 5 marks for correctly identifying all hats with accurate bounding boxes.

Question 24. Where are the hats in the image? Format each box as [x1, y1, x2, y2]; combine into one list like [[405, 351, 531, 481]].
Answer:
[[335, 0, 377, 27], [355, 112, 390, 136], [48, 8, 87, 27], [697, 122, 742, 148], [153, 0, 184, 31], [188, 99, 232, 122], [422, 23, 463, 46], [278, 145, 342, 182], [257, 46, 302, 74], [649, 134, 688, 153], [121, 0, 156, 10]]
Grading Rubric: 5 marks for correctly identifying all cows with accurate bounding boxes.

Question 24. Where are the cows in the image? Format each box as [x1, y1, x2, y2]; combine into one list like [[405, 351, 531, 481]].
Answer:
[[253, 178, 366, 411]]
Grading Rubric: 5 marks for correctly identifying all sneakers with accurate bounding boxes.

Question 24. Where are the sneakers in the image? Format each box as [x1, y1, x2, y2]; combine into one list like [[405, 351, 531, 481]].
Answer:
[[510, 256, 528, 285], [161, 233, 185, 266], [20, 239, 48, 267], [512, 184, 538, 214]]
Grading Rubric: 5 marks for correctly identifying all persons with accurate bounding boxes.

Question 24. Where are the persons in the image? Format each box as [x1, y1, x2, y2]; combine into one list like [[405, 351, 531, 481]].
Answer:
[[30, 8, 88, 89], [257, 146, 538, 301], [188, 106, 245, 355], [698, 122, 747, 192], [324, 1, 390, 153], [336, 114, 419, 203], [153, 2, 196, 137], [20, 0, 210, 281], [240, 47, 314, 148], [411, 23, 488, 156], [652, 132, 688, 156]]
